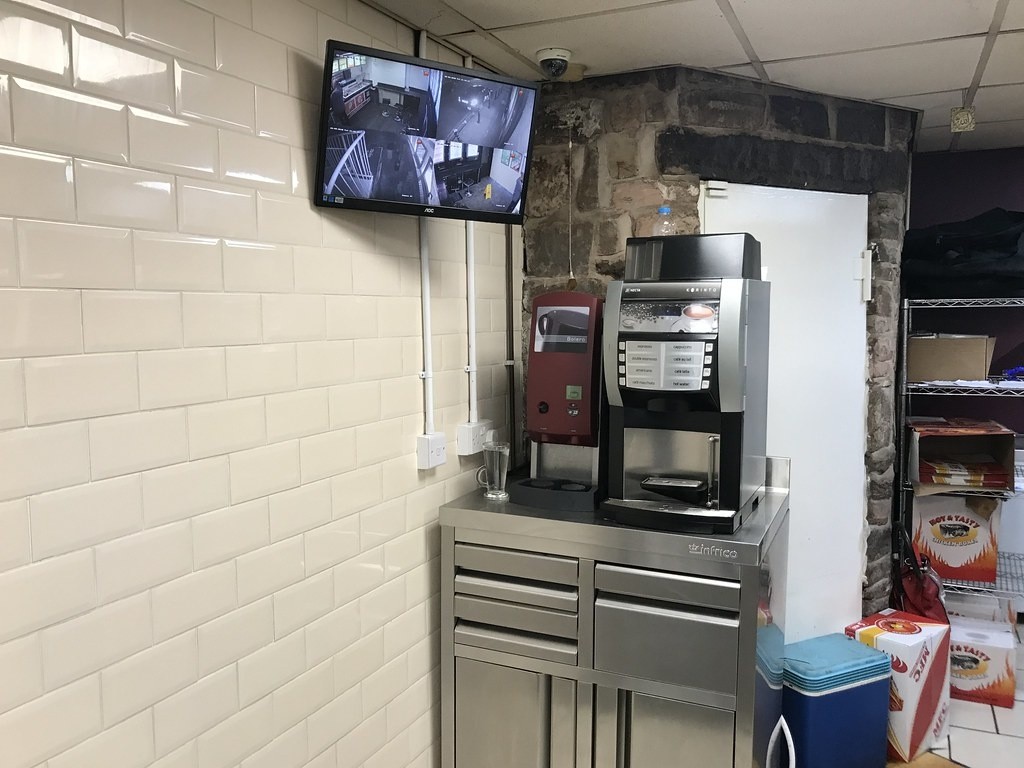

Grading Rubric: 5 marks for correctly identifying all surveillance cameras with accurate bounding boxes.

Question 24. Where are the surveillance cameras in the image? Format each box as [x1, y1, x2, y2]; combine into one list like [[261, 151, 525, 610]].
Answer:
[[537, 48, 571, 78]]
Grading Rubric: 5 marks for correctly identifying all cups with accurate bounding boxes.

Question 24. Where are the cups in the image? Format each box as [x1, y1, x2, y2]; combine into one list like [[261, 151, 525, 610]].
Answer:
[[474, 441, 511, 503]]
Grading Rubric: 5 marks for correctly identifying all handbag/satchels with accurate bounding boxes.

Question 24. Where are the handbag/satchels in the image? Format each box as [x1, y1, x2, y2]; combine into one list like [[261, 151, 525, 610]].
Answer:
[[901, 543, 950, 625]]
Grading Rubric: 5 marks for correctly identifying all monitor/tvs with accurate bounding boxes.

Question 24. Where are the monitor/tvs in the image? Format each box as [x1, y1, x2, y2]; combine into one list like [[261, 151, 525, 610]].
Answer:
[[314, 39, 542, 225]]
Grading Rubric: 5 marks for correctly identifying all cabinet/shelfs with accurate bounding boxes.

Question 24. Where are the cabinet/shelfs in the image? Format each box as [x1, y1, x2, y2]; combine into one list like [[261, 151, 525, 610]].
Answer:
[[894, 296, 1024, 617]]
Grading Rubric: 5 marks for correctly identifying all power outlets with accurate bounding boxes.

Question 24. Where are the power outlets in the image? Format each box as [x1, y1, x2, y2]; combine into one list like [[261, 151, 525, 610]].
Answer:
[[417, 431, 447, 468], [457, 417, 493, 455]]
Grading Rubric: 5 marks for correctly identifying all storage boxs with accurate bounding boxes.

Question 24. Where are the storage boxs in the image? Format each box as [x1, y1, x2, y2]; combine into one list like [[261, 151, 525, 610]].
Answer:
[[905, 414, 1015, 495], [845, 607, 952, 763], [781, 632, 893, 767], [907, 330, 996, 382], [911, 492, 998, 582], [948, 614, 1017, 708]]
